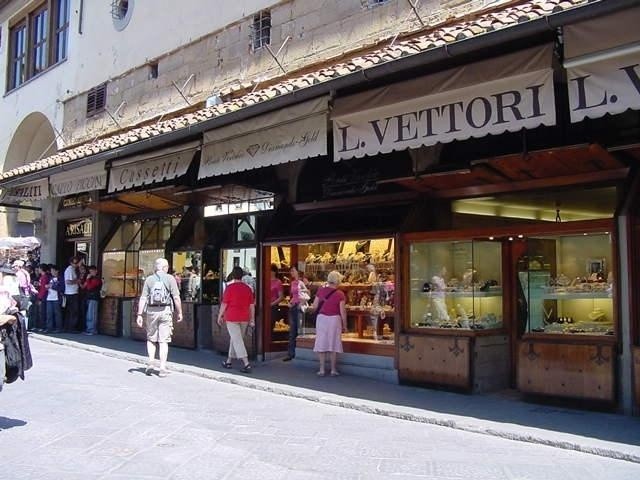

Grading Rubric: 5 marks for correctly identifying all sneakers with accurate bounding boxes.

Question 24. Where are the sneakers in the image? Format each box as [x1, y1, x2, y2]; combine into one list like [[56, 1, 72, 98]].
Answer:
[[30, 326, 98, 336], [144, 361, 157, 375], [283, 356, 295, 362], [158, 367, 172, 377]]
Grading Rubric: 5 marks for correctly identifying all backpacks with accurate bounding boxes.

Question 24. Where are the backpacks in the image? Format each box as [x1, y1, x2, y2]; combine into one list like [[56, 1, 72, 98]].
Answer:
[[149, 281, 170, 306]]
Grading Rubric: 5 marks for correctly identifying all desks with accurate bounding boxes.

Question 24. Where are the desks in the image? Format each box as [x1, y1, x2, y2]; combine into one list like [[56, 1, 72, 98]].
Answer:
[[346, 306, 394, 337]]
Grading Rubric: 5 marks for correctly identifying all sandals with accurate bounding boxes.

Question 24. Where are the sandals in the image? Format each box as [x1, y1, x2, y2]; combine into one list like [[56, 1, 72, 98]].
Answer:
[[221, 359, 232, 368], [240, 363, 252, 372]]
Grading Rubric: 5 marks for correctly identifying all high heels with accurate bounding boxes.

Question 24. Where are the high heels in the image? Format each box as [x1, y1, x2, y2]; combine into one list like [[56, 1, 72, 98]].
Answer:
[[331, 369, 341, 376], [317, 370, 326, 377]]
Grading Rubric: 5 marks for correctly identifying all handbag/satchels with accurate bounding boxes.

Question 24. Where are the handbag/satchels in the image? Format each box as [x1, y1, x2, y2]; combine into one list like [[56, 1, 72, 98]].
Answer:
[[312, 309, 319, 325], [61, 294, 66, 308]]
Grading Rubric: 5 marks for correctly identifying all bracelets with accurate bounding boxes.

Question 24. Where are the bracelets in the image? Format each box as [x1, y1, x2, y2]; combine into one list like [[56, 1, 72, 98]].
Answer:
[[136, 314, 143, 316]]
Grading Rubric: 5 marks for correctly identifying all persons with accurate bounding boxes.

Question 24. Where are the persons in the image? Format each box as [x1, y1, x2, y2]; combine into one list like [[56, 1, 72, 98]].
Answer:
[[282, 263, 309, 361], [430, 263, 450, 321], [242, 267, 256, 305], [451, 268, 479, 330], [135, 257, 183, 377], [271, 264, 284, 331], [172, 264, 201, 303], [0, 249, 103, 393], [304, 240, 396, 311], [216, 266, 254, 373], [305, 270, 348, 376]]
